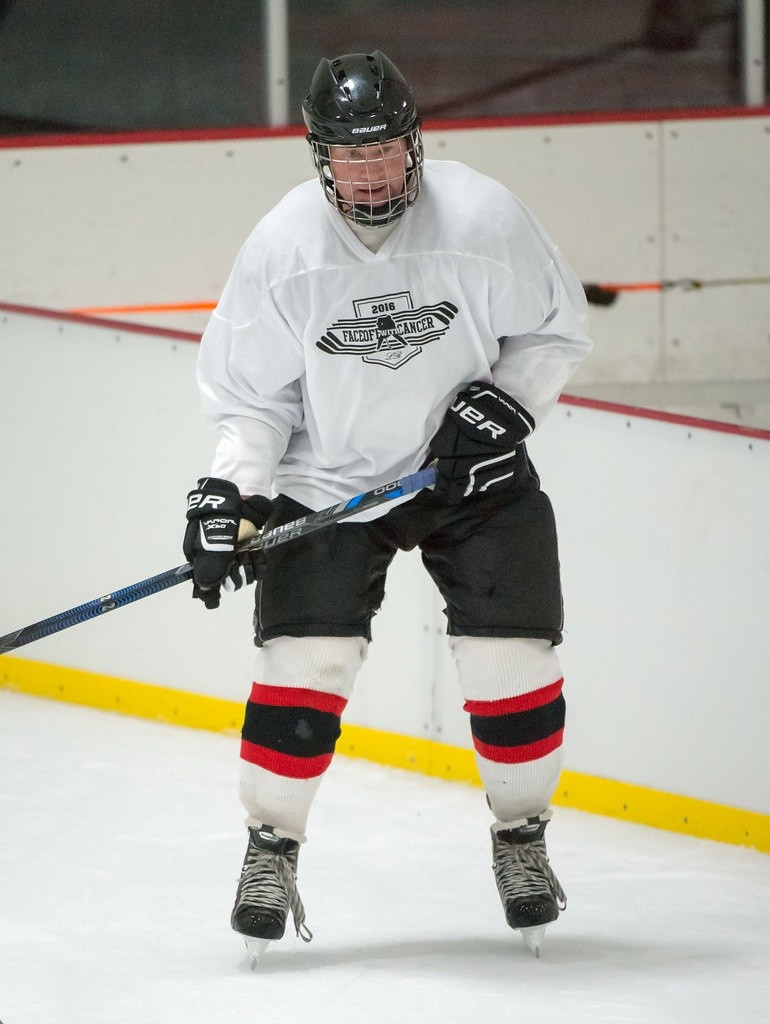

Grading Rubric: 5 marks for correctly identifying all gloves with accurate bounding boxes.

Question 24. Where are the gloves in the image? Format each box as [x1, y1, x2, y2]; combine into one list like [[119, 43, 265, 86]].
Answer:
[[183, 477, 275, 609], [415, 381, 540, 507]]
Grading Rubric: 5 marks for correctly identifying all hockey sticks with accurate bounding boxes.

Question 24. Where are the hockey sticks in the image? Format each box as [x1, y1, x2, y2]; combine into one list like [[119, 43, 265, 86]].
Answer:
[[1, 463, 439, 654]]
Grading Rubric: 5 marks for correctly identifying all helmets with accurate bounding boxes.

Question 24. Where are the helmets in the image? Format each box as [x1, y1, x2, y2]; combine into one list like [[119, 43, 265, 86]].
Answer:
[[301, 49, 425, 230]]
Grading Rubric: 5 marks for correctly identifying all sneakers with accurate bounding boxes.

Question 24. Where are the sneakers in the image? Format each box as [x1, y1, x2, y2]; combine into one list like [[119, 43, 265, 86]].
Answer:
[[489, 809, 567, 958], [230, 820, 314, 971]]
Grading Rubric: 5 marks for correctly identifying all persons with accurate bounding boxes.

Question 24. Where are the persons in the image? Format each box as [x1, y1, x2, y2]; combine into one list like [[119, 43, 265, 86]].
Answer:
[[183, 50, 591, 959]]
